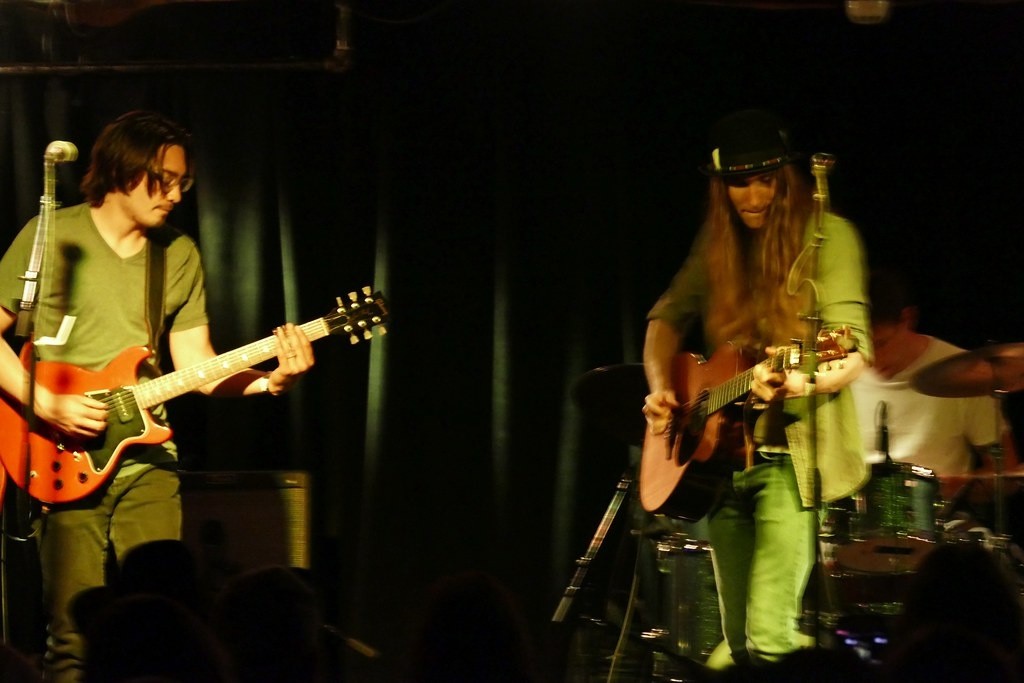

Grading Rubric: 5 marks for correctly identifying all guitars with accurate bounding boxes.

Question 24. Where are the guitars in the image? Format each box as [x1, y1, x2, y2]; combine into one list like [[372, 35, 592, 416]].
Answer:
[[0, 284, 394, 505], [640, 326, 858, 525]]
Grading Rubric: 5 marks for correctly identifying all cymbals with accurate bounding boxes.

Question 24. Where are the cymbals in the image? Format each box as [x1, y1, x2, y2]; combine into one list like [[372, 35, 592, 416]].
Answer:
[[909, 344, 1023, 397]]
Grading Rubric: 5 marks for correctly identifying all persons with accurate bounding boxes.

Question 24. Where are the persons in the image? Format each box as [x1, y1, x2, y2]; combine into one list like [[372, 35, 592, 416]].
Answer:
[[641, 115, 1024, 683], [0, 109, 315, 683]]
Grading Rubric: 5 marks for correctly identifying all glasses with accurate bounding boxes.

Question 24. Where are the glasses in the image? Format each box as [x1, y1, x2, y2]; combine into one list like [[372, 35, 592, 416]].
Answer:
[[141, 174, 194, 193]]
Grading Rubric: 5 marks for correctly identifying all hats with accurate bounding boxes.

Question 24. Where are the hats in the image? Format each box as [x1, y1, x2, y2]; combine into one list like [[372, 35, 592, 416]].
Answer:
[[699, 120, 802, 180]]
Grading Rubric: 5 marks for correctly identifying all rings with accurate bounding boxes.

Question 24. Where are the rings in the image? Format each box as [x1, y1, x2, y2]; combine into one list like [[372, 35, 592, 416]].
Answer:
[[286, 353, 296, 360]]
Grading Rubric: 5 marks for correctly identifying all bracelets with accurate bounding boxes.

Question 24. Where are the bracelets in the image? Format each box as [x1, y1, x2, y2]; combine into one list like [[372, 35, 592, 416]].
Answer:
[[261, 375, 268, 391]]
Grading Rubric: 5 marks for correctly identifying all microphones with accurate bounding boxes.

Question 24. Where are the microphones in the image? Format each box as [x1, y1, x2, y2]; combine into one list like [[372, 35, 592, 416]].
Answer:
[[43, 139, 78, 161], [876, 401, 891, 452], [808, 151, 836, 202]]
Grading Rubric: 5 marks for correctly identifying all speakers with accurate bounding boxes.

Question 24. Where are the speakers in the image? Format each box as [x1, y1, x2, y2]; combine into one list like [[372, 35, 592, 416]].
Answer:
[[174, 472, 312, 594]]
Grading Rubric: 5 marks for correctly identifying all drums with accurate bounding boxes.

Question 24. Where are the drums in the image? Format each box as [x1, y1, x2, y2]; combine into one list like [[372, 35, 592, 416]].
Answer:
[[654, 534, 723, 661], [825, 461, 944, 574]]
